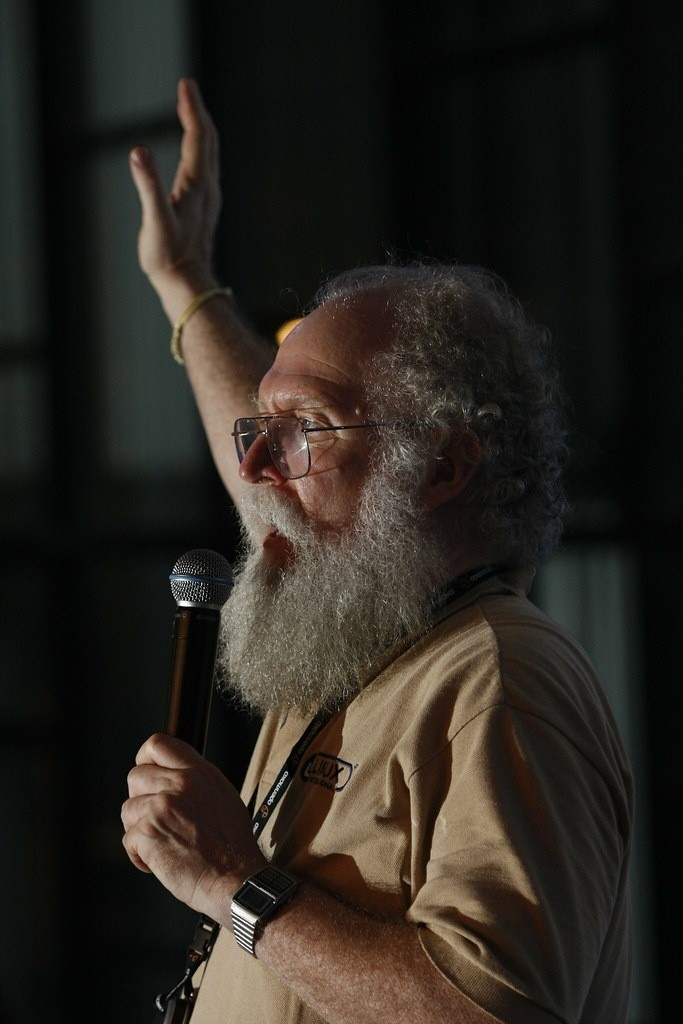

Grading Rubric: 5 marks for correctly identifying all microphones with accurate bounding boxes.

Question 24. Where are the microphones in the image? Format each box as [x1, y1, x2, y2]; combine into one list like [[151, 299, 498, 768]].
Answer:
[[162, 548, 236, 762]]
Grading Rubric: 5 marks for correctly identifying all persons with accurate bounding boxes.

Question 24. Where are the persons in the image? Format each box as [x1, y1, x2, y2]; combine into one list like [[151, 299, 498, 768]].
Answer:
[[122, 77, 637, 1024]]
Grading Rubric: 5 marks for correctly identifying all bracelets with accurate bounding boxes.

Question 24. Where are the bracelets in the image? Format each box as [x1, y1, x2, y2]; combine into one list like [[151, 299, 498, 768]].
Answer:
[[170, 287, 233, 364]]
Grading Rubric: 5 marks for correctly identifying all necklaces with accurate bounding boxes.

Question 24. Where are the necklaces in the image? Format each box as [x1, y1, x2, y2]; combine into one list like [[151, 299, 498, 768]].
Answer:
[[155, 565, 504, 1014]]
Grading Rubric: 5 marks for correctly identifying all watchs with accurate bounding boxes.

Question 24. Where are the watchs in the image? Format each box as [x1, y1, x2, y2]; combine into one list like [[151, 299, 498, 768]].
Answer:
[[230, 864, 302, 959]]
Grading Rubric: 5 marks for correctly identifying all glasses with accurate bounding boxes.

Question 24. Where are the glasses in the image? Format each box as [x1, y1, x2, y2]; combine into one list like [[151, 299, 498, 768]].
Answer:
[[232, 416, 417, 480]]
